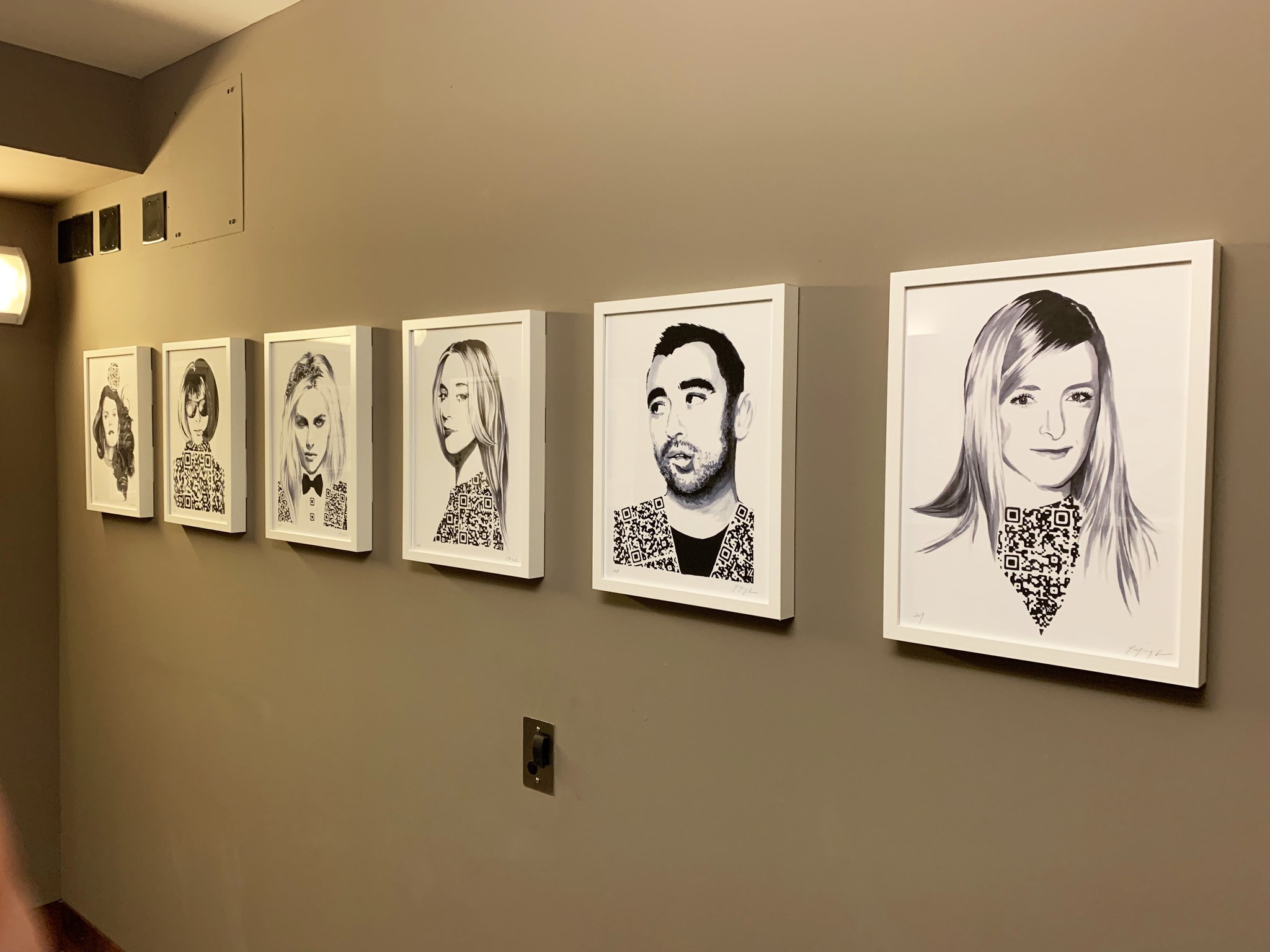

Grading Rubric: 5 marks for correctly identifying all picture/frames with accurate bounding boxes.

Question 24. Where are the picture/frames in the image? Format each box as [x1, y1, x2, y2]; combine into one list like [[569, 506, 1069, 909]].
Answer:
[[399, 308, 547, 583], [263, 322, 373, 553], [82, 345, 156, 519], [590, 281, 804, 622], [160, 335, 247, 536], [880, 237, 1226, 690]]
